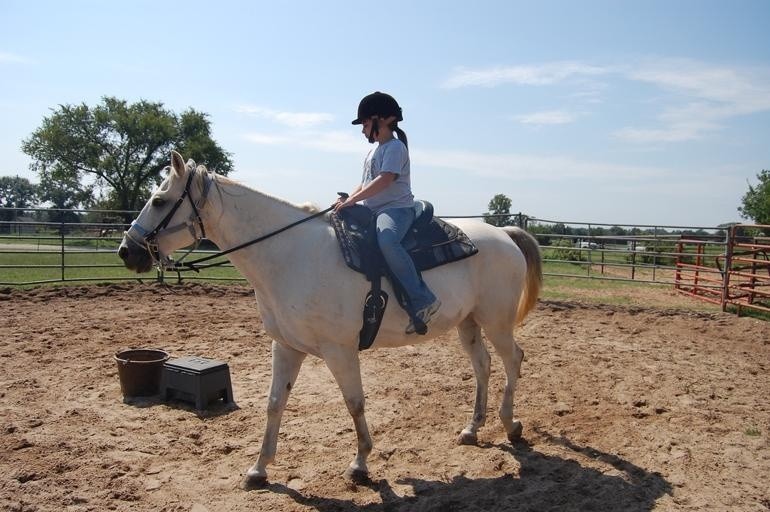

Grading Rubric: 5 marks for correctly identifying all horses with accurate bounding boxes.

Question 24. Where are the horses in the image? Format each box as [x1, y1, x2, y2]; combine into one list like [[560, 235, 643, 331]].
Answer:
[[117, 149, 545, 492]]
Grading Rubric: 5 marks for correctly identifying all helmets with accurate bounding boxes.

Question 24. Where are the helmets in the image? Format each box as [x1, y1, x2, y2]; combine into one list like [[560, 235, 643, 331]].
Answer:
[[351, 89, 404, 126]]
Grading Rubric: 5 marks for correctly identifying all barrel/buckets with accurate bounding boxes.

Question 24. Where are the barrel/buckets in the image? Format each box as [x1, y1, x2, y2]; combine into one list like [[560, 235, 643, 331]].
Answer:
[[113, 349, 170, 396]]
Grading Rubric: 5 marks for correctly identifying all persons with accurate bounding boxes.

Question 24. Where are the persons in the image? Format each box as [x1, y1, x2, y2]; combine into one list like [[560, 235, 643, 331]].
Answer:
[[332, 91, 441, 334]]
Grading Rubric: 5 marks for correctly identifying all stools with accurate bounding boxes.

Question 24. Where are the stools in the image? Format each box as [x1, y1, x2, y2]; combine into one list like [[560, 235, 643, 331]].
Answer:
[[158, 355, 234, 410]]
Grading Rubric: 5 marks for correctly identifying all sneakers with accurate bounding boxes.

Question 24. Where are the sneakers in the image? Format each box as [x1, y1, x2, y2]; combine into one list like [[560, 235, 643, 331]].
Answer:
[[404, 296, 448, 335]]
